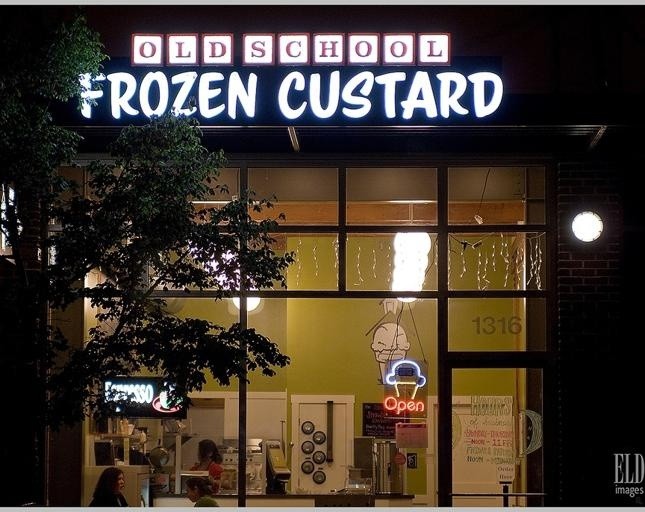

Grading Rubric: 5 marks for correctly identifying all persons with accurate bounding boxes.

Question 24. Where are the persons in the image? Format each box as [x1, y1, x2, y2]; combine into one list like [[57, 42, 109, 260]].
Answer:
[[192, 438, 223, 493], [184, 476, 220, 507], [88, 467, 130, 506]]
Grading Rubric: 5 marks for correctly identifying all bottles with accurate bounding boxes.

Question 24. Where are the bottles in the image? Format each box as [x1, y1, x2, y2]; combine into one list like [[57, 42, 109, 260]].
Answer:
[[107, 416, 128, 435]]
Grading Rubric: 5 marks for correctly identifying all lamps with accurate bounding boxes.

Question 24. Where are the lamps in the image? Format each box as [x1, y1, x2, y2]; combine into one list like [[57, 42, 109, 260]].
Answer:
[[570, 207, 605, 245], [188, 236, 261, 312], [391, 225, 432, 303]]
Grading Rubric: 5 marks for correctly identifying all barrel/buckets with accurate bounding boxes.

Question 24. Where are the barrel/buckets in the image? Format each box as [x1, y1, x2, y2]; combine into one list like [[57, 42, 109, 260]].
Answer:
[[372, 442, 405, 493]]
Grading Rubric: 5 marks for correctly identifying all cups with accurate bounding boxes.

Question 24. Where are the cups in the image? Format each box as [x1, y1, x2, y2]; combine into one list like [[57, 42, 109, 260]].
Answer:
[[128, 424, 134, 435], [160, 391, 170, 409]]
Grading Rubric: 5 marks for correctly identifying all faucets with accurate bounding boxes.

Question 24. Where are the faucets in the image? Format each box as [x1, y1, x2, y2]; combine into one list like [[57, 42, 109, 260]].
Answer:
[[388, 463, 392, 474]]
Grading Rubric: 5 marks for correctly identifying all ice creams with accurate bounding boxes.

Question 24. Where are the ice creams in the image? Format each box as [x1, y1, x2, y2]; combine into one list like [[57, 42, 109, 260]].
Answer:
[[371, 322, 410, 384], [386, 360, 426, 400]]
